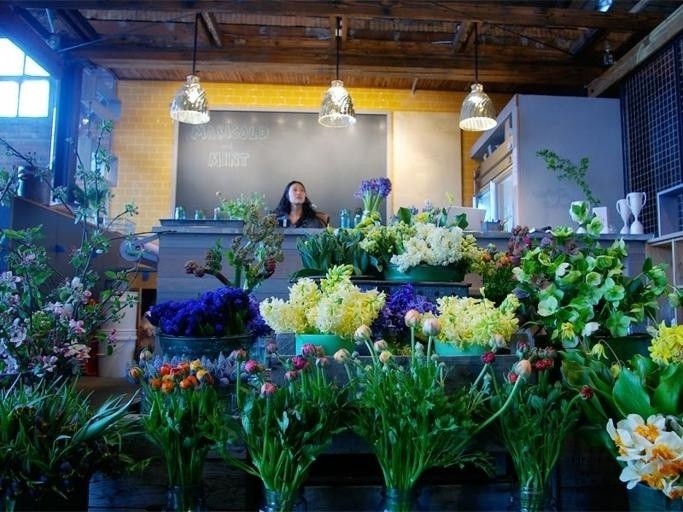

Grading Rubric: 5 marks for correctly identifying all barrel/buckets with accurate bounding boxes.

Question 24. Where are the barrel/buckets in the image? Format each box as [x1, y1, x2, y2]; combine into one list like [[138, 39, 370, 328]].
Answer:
[[93, 330, 137, 378], [17, 165, 51, 207], [101, 292, 139, 330]]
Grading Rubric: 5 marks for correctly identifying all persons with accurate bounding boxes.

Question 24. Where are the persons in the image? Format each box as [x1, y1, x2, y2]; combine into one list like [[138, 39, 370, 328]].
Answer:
[[270, 181, 321, 228]]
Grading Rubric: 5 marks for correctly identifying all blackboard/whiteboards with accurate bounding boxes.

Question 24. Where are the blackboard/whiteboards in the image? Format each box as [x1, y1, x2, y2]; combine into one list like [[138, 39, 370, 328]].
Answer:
[[170, 106, 393, 226]]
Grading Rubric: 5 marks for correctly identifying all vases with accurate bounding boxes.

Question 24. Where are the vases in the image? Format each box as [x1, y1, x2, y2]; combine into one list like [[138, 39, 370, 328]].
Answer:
[[164, 482, 562, 511]]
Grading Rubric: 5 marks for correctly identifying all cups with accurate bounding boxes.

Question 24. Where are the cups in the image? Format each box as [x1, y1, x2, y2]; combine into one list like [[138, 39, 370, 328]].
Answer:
[[173, 206, 186, 220]]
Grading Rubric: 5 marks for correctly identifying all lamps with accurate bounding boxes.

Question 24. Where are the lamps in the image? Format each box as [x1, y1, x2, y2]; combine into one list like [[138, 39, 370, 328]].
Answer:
[[318, 14, 357, 129], [170, 12, 211, 125], [457, 23, 497, 132]]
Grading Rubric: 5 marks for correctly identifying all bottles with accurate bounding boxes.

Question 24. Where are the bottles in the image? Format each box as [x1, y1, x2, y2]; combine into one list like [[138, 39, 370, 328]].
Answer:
[[213, 207, 224, 220], [353, 208, 362, 228], [195, 209, 206, 219], [338, 208, 351, 229]]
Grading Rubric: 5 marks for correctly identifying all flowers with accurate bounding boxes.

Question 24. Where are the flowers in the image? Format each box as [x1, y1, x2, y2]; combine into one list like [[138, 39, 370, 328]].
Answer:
[[1, 97, 683, 503]]
